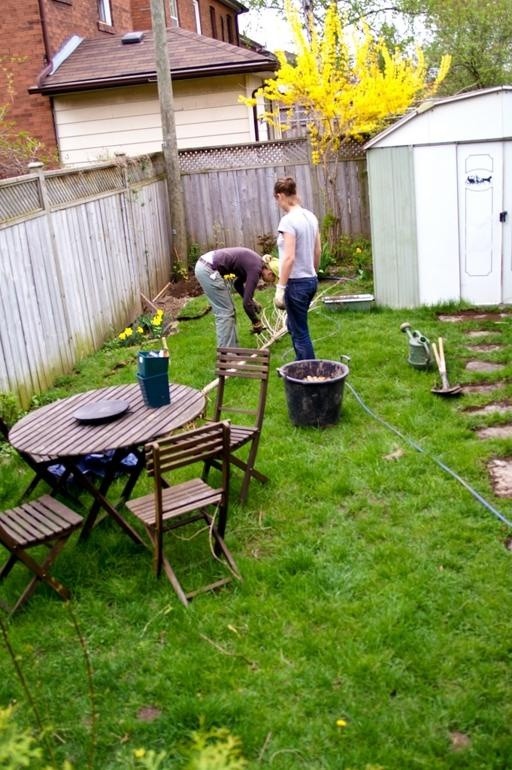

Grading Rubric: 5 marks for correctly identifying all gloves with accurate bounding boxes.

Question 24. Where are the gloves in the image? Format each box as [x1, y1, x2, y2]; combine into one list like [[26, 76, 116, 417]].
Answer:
[[252, 298, 262, 314], [275, 284, 287, 310], [249, 320, 268, 335]]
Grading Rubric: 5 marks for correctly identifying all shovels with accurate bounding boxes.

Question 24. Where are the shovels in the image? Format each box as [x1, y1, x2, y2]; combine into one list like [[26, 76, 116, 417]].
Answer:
[[431, 342, 462, 394], [431, 337, 460, 394]]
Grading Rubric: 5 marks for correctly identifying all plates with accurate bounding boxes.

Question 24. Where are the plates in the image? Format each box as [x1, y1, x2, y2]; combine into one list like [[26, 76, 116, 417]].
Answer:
[[74, 400, 129, 419]]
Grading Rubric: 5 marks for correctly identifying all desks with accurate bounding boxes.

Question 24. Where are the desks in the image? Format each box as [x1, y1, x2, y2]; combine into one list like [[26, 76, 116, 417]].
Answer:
[[8, 383, 206, 548]]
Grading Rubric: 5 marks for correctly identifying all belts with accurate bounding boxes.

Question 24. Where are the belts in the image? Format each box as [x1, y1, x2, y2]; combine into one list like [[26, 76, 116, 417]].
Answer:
[[199, 257, 213, 270]]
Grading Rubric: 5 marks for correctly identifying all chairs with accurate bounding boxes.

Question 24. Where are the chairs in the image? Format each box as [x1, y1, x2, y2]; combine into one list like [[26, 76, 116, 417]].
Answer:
[[125, 418, 244, 607], [0, 494, 84, 619], [1, 417, 85, 510], [173, 347, 270, 503]]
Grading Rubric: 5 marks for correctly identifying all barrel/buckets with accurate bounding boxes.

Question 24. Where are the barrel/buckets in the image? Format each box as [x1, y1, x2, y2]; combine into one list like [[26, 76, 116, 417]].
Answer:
[[275, 358, 350, 432]]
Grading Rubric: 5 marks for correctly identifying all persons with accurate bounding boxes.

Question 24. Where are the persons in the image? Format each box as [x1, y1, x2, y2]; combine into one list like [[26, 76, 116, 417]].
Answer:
[[273, 177, 322, 361], [194, 246, 280, 357]]
